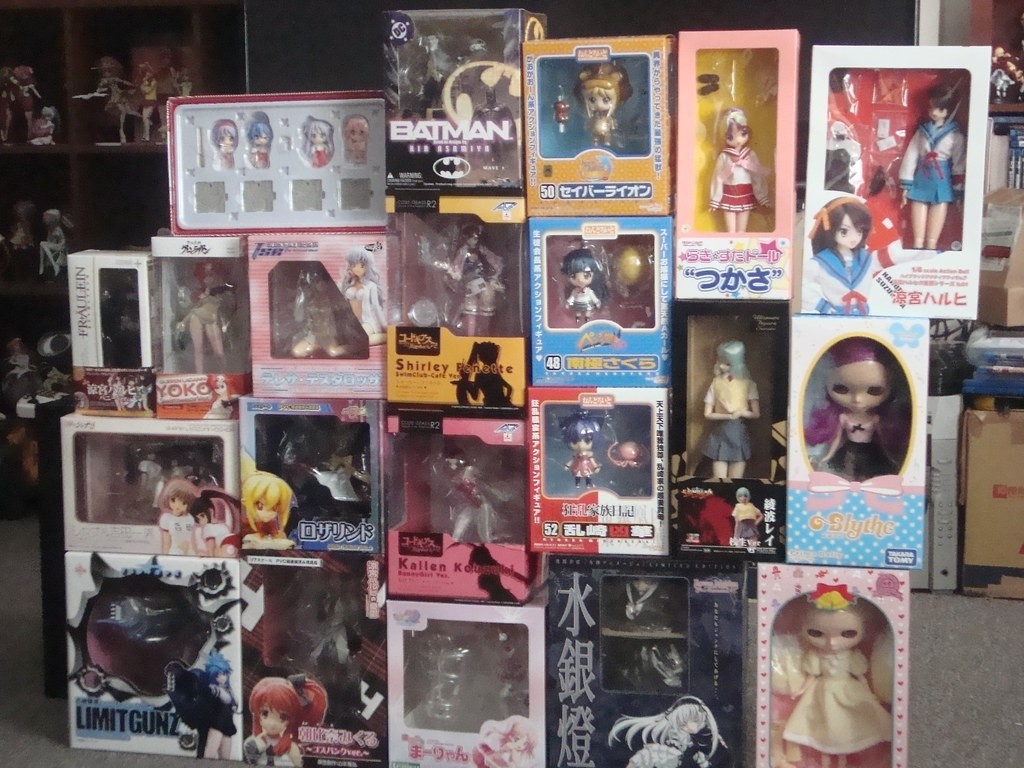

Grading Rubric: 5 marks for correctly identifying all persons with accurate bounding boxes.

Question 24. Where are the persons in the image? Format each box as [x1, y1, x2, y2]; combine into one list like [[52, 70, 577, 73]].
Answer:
[[1, 336, 44, 400], [444, 448, 511, 542], [134, 444, 222, 524], [0, 64, 58, 146], [574, 61, 633, 147], [818, 336, 903, 484], [991, 45, 1024, 104], [2, 197, 74, 278], [442, 219, 504, 337], [699, 338, 761, 482], [603, 572, 691, 698], [343, 114, 369, 165], [559, 247, 608, 323], [290, 267, 360, 359], [898, 81, 965, 251], [176, 257, 239, 375], [781, 595, 894, 768], [304, 119, 334, 166], [94, 54, 194, 142], [212, 121, 240, 167], [706, 106, 773, 234], [247, 122, 271, 167]]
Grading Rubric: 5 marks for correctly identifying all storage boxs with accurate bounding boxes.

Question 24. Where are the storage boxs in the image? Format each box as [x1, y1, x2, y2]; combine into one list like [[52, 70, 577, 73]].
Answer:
[[527, 386, 668, 556], [387, 586, 546, 768], [249, 233, 383, 396], [152, 232, 250, 419], [757, 562, 911, 768], [386, 196, 526, 413], [164, 92, 388, 233], [528, 215, 674, 387], [669, 299, 791, 563], [241, 551, 385, 768], [383, 8, 548, 196], [239, 397, 386, 554], [521, 34, 672, 216], [800, 42, 993, 319], [67, 248, 157, 418], [677, 29, 793, 301], [383, 404, 547, 606], [546, 556, 748, 768], [60, 412, 242, 761], [789, 314, 1024, 599]]
